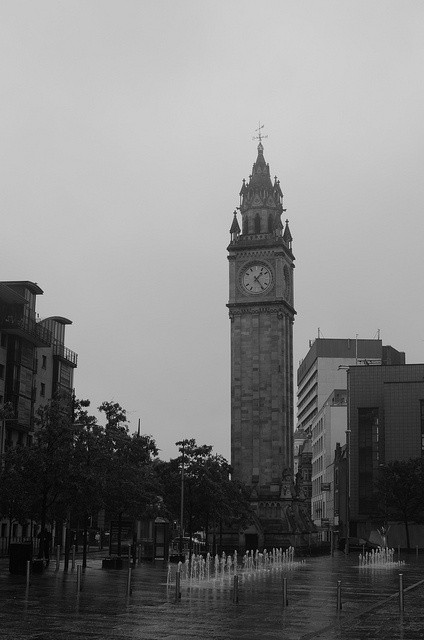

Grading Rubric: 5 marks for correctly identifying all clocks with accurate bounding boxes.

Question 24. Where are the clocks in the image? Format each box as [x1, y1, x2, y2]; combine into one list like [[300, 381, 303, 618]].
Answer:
[[237, 259, 275, 296]]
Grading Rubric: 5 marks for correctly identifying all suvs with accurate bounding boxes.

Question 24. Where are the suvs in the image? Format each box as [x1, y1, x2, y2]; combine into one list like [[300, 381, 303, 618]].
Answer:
[[171, 536, 203, 556]]
